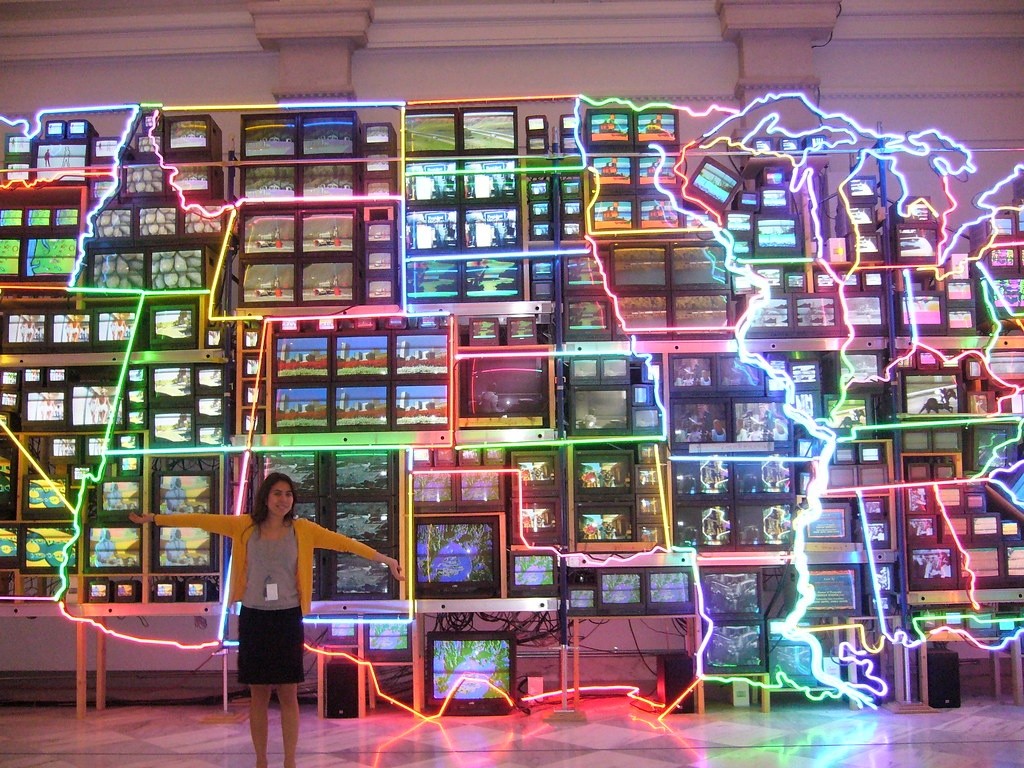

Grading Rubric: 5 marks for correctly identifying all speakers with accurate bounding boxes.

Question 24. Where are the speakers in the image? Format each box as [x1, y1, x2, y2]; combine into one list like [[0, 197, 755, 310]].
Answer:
[[916, 648, 961, 708], [325, 662, 358, 720], [655, 653, 694, 714], [840, 647, 882, 706]]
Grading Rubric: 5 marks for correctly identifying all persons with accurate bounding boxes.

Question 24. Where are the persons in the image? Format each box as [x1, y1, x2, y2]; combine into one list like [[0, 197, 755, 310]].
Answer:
[[166, 477, 194, 513], [130, 472, 406, 768], [107, 484, 127, 510], [699, 370, 711, 386], [95, 529, 124, 567], [164, 528, 195, 565], [710, 419, 726, 441]]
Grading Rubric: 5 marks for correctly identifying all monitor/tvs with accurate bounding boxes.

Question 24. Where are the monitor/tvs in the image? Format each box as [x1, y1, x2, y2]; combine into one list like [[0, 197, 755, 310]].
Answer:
[[0, 102, 1024, 717]]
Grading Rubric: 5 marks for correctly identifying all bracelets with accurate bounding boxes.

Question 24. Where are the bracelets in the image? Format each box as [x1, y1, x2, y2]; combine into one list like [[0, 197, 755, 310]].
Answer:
[[153, 513, 156, 522]]
